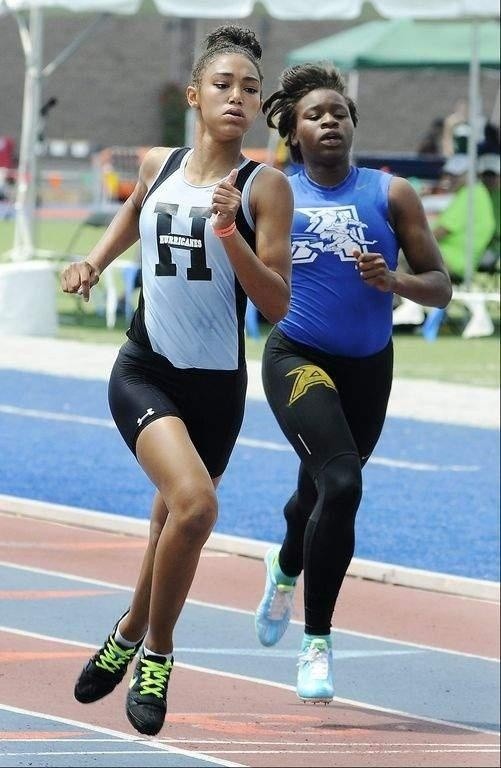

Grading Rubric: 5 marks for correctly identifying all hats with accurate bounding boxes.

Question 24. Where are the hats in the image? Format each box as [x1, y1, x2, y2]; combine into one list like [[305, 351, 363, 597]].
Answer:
[[441, 154, 468, 176], [477, 153, 500, 176]]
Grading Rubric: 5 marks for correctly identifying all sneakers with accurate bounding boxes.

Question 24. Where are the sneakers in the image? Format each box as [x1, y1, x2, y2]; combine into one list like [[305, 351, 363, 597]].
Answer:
[[126, 651, 176, 735], [256, 549, 297, 646], [296, 640, 336, 705], [463, 313, 494, 338], [391, 300, 424, 325], [74, 612, 144, 704]]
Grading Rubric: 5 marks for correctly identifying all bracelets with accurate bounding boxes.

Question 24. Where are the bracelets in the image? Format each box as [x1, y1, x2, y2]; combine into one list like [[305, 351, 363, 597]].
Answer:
[[213, 221, 238, 239]]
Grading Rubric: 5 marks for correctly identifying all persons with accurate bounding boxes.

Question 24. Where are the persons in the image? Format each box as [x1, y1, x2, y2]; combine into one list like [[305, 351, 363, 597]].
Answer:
[[422, 112, 501, 288], [256, 68, 454, 700], [61, 27, 296, 738]]
